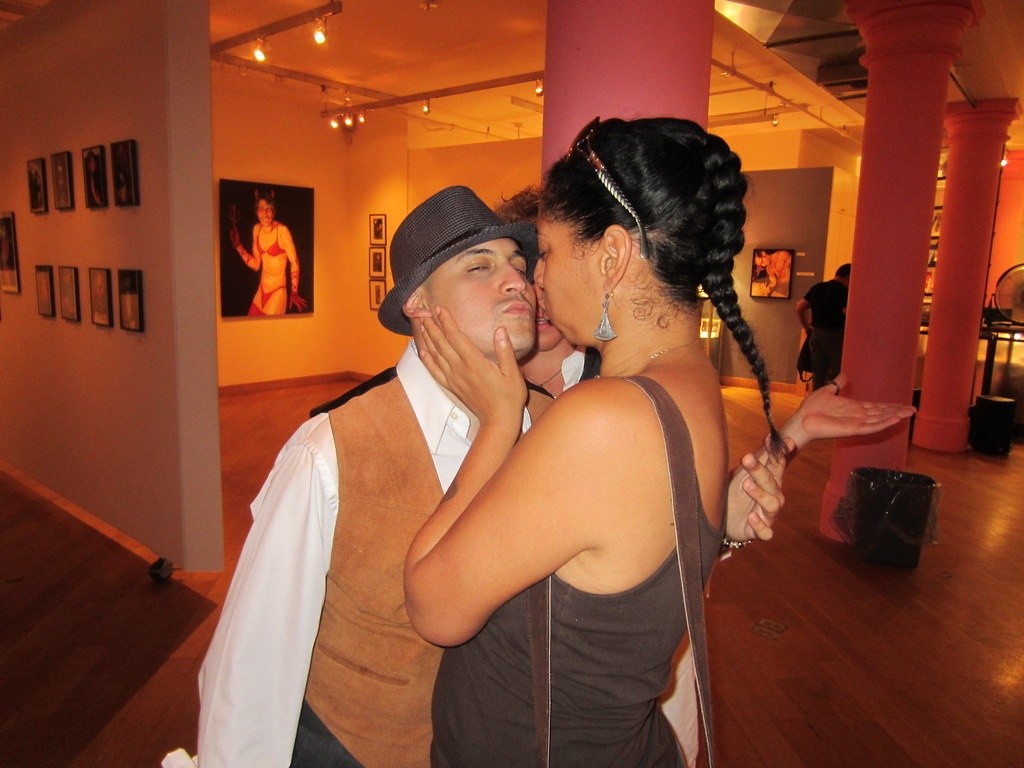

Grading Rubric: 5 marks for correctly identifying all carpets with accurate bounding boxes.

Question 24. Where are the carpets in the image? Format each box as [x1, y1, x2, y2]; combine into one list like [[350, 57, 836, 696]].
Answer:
[[0, 468, 218, 767]]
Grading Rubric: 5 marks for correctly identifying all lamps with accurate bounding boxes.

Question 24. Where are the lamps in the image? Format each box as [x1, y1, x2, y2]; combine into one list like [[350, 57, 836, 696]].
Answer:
[[770, 113, 778, 125], [212, 0, 543, 130]]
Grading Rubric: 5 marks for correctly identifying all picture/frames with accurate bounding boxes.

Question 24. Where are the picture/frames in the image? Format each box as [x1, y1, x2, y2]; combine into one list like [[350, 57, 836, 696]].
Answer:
[[0, 138, 144, 332], [370, 214, 386, 310], [924, 177, 947, 296], [749, 248, 795, 299]]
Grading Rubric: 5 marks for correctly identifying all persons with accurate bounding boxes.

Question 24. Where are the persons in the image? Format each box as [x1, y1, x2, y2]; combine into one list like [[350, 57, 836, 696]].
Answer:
[[160, 184, 787, 767], [490, 187, 921, 768], [226, 186, 307, 318], [752, 249, 792, 297], [795, 263, 851, 390], [400, 121, 783, 768]]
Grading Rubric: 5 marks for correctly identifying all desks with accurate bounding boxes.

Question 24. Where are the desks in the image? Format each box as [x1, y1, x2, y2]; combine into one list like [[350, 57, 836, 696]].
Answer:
[[920, 319, 1024, 420]]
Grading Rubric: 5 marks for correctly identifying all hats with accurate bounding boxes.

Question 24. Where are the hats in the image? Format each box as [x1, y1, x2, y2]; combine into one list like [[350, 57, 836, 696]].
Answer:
[[377, 185, 537, 335]]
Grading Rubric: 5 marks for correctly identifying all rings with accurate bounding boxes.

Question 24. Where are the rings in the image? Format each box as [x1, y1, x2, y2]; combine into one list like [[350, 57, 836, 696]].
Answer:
[[829, 380, 841, 395]]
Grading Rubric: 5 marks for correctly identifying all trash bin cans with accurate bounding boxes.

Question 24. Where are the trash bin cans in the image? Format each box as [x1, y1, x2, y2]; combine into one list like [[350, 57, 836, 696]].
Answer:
[[852, 468, 936, 568]]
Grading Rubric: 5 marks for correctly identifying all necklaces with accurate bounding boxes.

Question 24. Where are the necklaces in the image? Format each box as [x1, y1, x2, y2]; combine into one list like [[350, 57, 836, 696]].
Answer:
[[609, 341, 697, 379], [525, 366, 563, 399]]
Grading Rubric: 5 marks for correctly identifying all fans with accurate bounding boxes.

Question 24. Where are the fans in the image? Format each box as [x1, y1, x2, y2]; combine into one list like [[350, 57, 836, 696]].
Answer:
[[993, 263, 1024, 326]]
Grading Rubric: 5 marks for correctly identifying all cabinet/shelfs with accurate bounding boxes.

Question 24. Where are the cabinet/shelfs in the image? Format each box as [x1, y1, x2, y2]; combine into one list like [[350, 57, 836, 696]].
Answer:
[[695, 284, 724, 377]]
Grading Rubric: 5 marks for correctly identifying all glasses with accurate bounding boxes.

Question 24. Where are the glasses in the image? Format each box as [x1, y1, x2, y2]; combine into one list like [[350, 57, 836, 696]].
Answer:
[[567, 115, 651, 263]]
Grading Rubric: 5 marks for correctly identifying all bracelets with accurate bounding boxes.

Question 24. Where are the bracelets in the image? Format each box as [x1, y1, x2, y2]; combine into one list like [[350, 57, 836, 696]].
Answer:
[[721, 538, 751, 549]]
